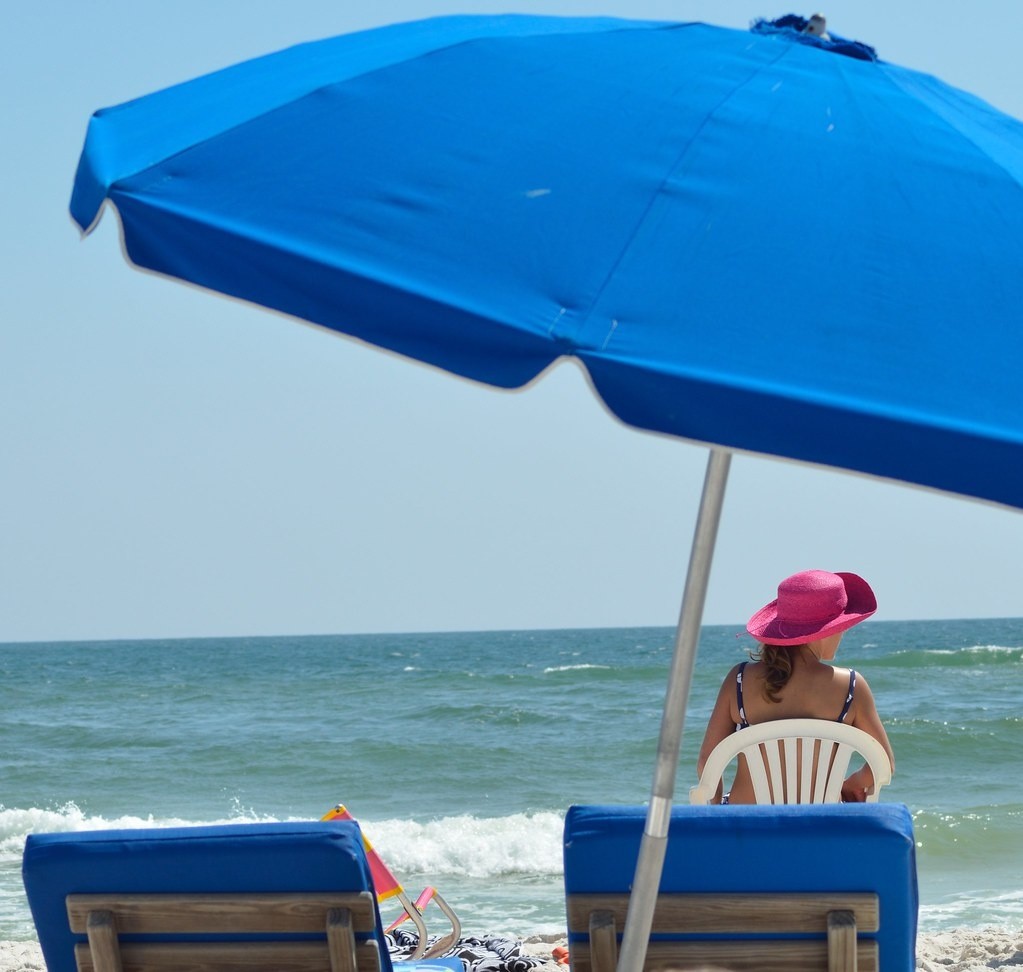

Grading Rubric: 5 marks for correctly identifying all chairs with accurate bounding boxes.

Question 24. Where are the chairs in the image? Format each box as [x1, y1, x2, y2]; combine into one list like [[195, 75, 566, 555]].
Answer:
[[689, 717, 892, 810], [561, 803, 919, 972], [20, 818, 393, 972]]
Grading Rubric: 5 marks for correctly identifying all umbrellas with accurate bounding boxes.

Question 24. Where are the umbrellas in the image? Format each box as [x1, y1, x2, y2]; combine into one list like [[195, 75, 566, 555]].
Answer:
[[69, 14, 1023, 972]]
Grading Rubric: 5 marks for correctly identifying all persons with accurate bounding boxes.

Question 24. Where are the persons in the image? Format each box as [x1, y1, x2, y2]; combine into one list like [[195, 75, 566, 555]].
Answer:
[[697, 569, 895, 805]]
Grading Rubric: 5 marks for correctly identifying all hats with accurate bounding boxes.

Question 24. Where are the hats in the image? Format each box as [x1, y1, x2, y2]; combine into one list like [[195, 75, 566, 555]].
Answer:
[[744, 570, 878, 645]]
